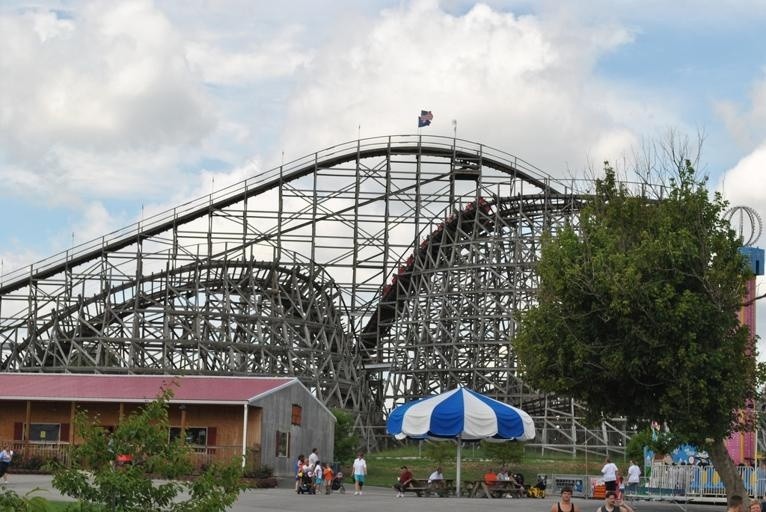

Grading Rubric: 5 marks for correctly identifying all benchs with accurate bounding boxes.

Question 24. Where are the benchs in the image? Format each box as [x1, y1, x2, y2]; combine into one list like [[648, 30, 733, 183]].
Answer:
[[405, 479, 524, 498]]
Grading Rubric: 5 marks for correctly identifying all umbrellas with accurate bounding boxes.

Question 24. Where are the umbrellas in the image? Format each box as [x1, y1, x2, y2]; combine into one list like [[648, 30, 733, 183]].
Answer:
[[387, 387, 536, 497]]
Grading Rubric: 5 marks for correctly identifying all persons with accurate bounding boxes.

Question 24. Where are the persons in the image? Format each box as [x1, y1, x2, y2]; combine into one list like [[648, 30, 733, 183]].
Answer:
[[350, 453, 367, 495], [485, 467, 547, 498], [550, 457, 766, 511], [394, 466, 413, 498], [0, 445, 12, 483], [428, 467, 443, 497], [295, 448, 334, 495]]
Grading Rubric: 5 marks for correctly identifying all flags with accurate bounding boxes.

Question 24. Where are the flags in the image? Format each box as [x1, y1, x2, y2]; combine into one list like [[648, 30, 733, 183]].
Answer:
[[419, 117, 431, 126], [421, 110, 433, 119]]
